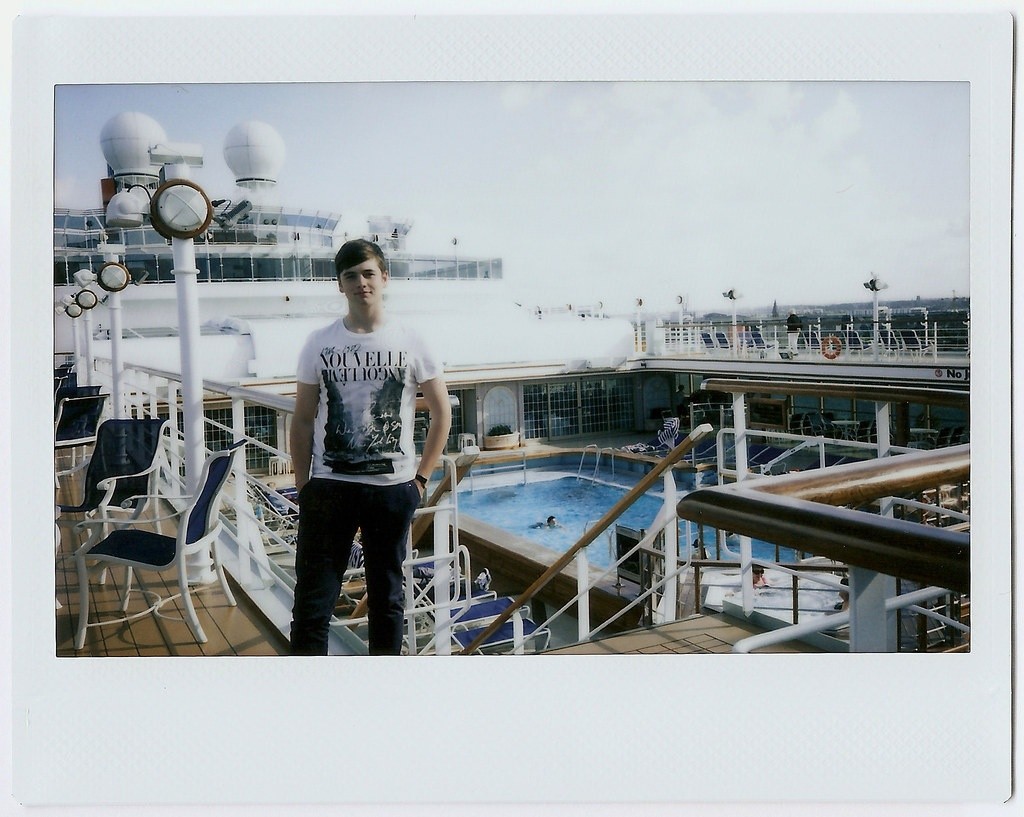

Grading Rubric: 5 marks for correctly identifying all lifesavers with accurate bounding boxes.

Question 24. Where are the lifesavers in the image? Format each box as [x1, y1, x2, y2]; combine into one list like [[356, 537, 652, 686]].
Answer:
[[820, 336, 842, 360]]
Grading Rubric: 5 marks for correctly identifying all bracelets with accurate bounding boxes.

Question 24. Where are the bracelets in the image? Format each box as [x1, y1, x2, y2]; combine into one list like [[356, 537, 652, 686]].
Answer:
[[413, 473, 427, 489]]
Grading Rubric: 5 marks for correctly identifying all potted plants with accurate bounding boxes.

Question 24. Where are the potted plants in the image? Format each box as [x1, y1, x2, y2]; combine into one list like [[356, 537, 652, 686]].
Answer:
[[482, 424, 520, 448]]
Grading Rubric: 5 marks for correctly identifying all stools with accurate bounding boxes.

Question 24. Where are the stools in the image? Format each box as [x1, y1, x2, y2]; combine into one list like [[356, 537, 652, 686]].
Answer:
[[268, 456, 290, 476], [457, 432, 475, 451]]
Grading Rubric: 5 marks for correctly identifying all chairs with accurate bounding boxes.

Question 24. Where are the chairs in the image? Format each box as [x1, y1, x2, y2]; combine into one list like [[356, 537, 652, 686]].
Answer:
[[621, 402, 965, 473], [701, 326, 939, 363], [219, 471, 552, 656], [55, 362, 111, 490], [55, 418, 176, 583], [76, 437, 251, 644]]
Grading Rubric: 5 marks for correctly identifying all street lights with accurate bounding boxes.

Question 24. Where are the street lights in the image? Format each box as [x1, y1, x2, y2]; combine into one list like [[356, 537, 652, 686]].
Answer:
[[634, 297, 644, 353], [54, 168, 131, 493], [863, 270, 890, 357], [721, 285, 743, 359], [102, 110, 285, 583], [675, 292, 686, 348]]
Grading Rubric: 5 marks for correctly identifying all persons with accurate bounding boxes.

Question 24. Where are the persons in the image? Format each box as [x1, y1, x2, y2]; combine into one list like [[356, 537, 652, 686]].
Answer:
[[724, 565, 770, 597], [529, 516, 564, 531], [286, 237, 452, 655], [838, 577, 849, 612], [675, 385, 685, 419], [691, 538, 711, 560], [785, 309, 802, 355]]
